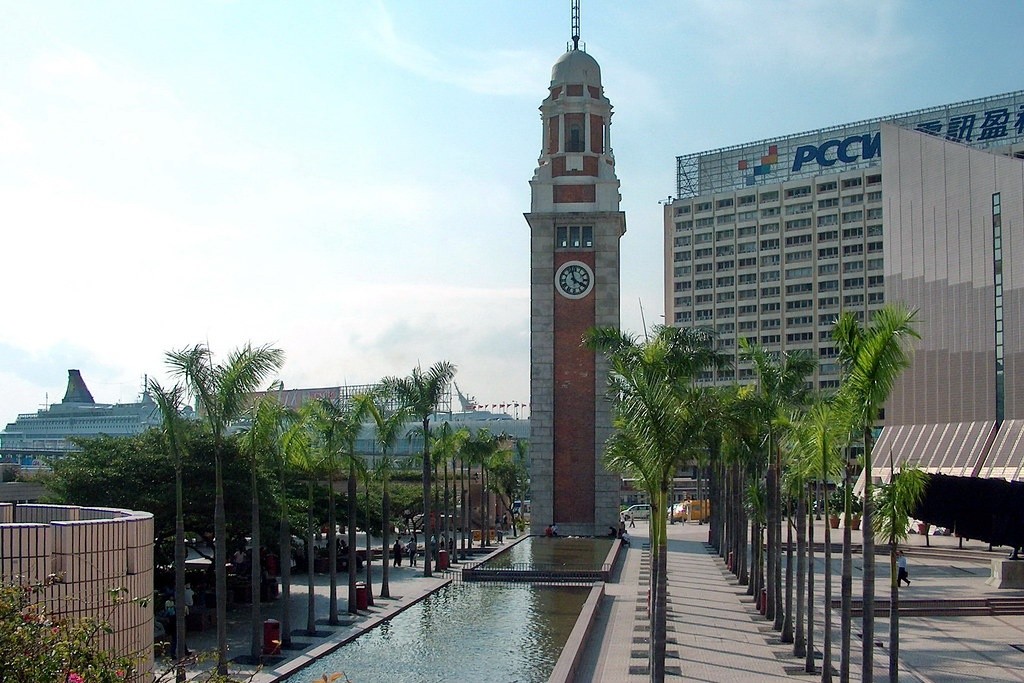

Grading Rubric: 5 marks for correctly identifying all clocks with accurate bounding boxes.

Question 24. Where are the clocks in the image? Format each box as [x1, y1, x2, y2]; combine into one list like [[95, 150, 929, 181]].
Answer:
[[559, 266, 590, 295]]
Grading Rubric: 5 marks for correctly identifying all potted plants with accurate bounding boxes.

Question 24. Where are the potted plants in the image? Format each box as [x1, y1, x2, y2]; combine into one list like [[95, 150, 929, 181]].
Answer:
[[829, 486, 862, 530]]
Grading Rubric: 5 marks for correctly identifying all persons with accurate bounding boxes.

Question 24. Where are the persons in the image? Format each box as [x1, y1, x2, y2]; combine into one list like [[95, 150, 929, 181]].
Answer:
[[234, 551, 246, 576], [336, 538, 346, 553], [622, 530, 631, 547], [430, 533, 453, 561], [621, 513, 625, 524], [392, 536, 405, 567], [629, 512, 635, 528], [897, 550, 910, 588], [502, 512, 507, 524], [164, 582, 195, 660], [408, 537, 417, 567], [608, 526, 617, 539], [546, 522, 560, 537], [495, 515, 502, 523], [314, 546, 319, 559]]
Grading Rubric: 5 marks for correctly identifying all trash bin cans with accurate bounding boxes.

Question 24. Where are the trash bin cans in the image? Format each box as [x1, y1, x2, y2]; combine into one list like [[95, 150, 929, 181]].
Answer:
[[439, 550, 448, 570], [265, 619, 281, 654], [356, 582, 368, 611], [728, 552, 733, 571], [497, 531, 503, 543], [760, 588, 767, 614]]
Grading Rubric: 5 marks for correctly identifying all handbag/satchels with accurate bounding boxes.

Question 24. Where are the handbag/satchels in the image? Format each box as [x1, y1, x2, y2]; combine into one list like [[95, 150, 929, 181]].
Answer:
[[905, 571, 909, 578]]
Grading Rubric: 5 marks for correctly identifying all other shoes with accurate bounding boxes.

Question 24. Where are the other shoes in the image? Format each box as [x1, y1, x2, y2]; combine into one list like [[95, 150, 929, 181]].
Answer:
[[633, 527, 635, 528], [906, 580, 911, 588], [629, 526, 631, 527], [410, 563, 412, 567], [398, 564, 401, 566], [393, 565, 395, 567]]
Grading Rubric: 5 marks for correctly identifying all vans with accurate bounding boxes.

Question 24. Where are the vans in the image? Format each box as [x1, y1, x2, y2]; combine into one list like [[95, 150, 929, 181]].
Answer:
[[621, 504, 651, 519], [669, 500, 710, 523], [502, 501, 530, 523]]
